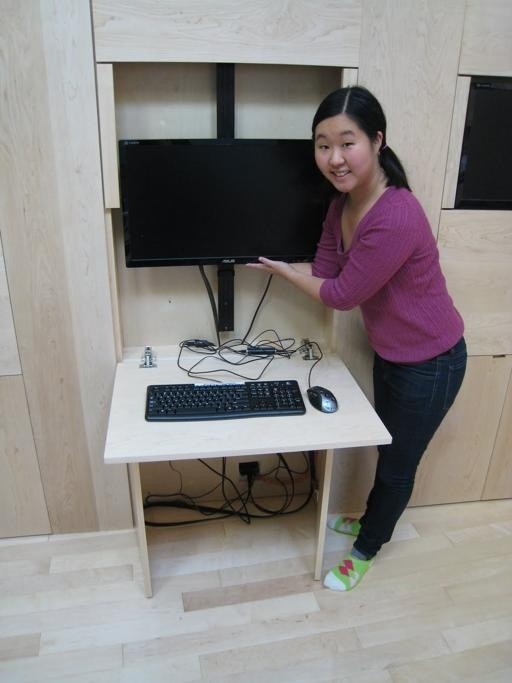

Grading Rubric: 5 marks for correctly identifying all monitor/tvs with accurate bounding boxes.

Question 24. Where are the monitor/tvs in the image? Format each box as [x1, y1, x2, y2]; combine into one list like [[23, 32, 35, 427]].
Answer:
[[453, 77, 512, 210], [117, 138, 335, 268]]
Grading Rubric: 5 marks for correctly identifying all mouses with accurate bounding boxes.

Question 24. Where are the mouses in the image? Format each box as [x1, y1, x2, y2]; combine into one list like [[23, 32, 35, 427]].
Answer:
[[307, 386, 338, 413]]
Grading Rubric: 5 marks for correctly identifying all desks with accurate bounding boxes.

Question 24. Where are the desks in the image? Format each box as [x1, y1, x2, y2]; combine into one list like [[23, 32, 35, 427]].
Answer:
[[103, 338, 394, 600]]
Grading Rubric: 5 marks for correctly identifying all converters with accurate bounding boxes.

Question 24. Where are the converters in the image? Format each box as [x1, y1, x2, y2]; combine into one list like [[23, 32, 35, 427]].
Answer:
[[246, 346, 276, 355]]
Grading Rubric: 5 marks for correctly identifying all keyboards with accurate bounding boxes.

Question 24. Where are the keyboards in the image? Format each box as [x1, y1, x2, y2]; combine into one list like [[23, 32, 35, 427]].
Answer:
[[145, 380, 306, 421]]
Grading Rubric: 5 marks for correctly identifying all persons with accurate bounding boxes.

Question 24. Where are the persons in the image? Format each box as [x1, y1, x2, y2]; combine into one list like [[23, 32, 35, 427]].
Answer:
[[248, 85, 468, 594]]
